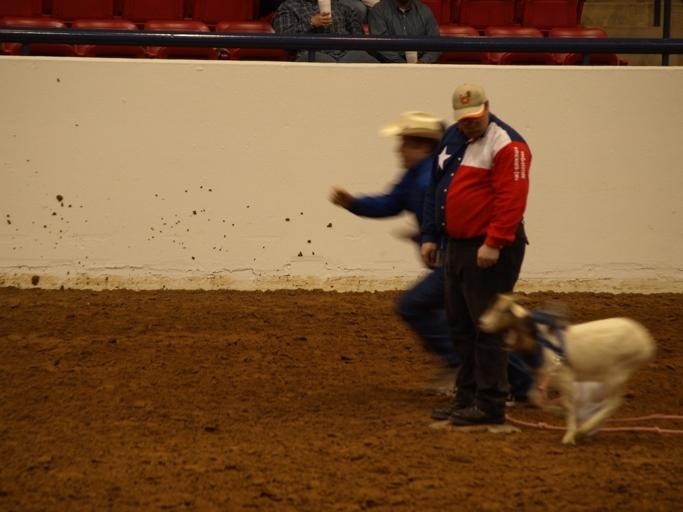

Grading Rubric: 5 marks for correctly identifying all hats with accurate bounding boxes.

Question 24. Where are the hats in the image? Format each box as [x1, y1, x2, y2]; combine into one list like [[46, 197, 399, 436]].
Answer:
[[383, 110, 446, 140], [452, 83, 489, 121]]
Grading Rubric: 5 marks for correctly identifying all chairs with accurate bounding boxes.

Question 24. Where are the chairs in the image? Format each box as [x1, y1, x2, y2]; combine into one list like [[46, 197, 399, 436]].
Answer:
[[0, 0, 629, 65]]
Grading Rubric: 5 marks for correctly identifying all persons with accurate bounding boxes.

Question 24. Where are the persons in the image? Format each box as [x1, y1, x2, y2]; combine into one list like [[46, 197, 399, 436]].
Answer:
[[429, 82, 533, 427], [367, 1, 446, 65], [327, 111, 518, 408], [271, 1, 379, 62]]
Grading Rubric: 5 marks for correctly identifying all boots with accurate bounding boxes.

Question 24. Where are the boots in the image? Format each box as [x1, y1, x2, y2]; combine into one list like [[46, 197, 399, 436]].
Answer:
[[432, 375, 475, 420], [452, 394, 505, 426]]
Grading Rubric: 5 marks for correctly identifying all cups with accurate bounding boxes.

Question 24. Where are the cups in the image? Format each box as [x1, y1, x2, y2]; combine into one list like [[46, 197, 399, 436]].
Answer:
[[405, 50, 418, 63], [318, 0, 331, 18]]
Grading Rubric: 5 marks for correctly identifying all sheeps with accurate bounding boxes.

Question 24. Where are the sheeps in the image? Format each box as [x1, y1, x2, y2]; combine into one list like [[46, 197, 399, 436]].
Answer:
[[475, 292, 659, 448]]
